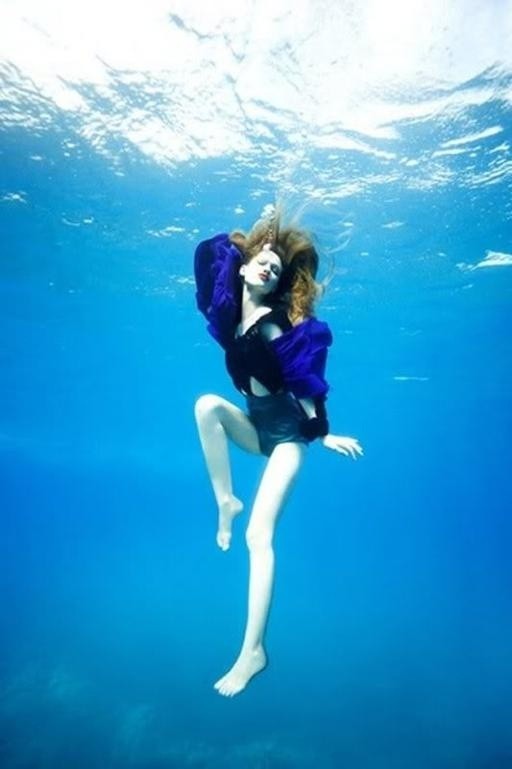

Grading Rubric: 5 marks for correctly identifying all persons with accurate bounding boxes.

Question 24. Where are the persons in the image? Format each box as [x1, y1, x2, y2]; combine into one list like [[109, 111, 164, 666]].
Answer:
[[192, 202, 365, 700]]
